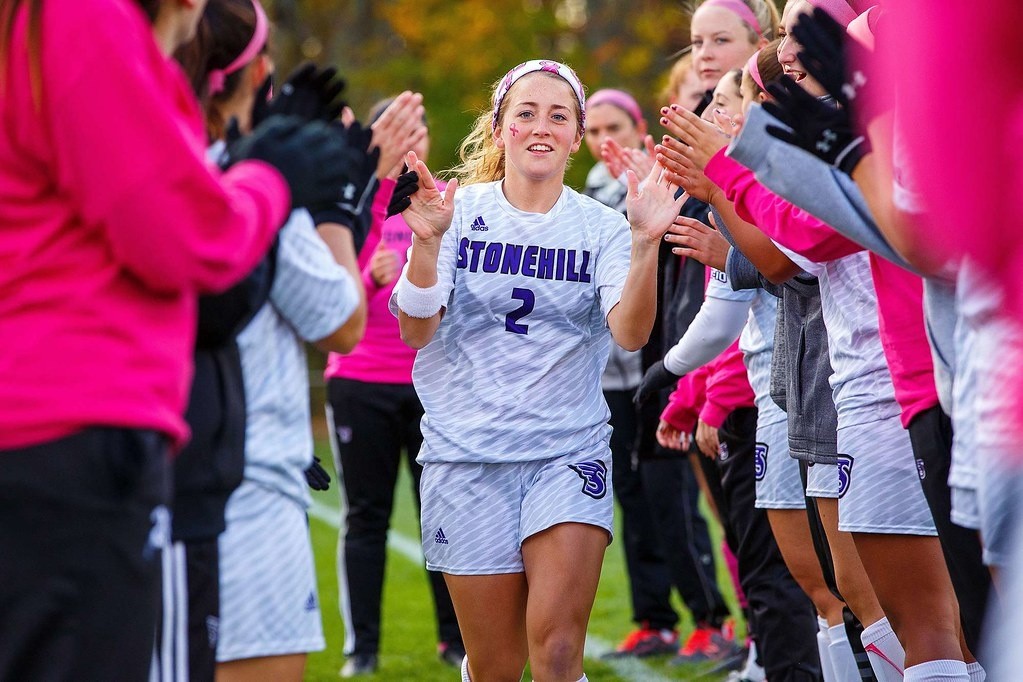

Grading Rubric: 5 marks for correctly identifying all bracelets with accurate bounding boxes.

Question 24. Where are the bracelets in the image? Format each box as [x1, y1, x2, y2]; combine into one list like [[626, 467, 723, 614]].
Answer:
[[397, 270, 443, 320]]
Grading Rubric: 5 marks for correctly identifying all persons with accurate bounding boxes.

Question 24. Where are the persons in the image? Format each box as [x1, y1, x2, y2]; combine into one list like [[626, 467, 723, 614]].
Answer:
[[574, 88, 739, 666], [388, 60, 691, 682], [324, 93, 466, 680], [0, 0, 427, 682], [601, 0, 1023, 682]]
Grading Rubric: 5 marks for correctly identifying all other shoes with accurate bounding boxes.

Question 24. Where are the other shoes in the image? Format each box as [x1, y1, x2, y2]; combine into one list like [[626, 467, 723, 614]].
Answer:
[[666, 618, 739, 666], [601, 620, 679, 661], [438, 641, 466, 669], [340, 655, 378, 678]]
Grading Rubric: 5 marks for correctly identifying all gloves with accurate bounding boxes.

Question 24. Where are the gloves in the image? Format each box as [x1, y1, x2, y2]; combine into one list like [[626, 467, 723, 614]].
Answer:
[[222, 60, 419, 258], [762, 74, 872, 180], [303, 457, 331, 492], [630, 416, 665, 472], [632, 359, 686, 405], [793, 6, 886, 124]]
